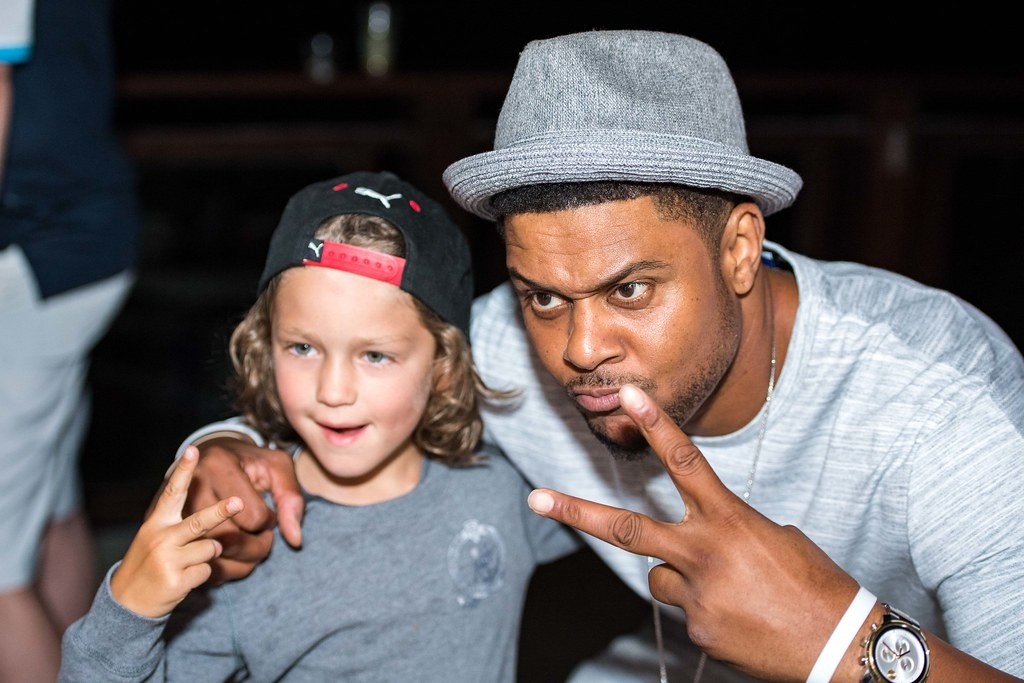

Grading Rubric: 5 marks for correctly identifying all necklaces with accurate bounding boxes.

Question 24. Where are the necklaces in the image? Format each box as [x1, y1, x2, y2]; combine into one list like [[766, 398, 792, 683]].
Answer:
[[597, 264, 803, 683]]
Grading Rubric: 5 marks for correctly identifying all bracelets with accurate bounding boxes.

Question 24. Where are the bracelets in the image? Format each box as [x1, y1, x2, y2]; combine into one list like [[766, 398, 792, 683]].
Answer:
[[805, 585, 878, 683]]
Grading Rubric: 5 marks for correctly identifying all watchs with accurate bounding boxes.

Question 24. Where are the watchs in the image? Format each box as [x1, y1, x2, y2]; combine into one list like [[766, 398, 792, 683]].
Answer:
[[859, 595, 934, 683]]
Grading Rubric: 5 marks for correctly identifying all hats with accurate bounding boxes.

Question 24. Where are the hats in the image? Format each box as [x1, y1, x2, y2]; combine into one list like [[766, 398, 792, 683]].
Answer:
[[441, 30, 805, 217], [255, 172, 476, 335]]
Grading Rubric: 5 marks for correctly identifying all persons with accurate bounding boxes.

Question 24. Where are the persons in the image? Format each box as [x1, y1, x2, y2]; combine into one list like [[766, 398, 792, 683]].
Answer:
[[0, 0, 141, 683], [63, 170, 582, 683], [146, 31, 1024, 683]]
